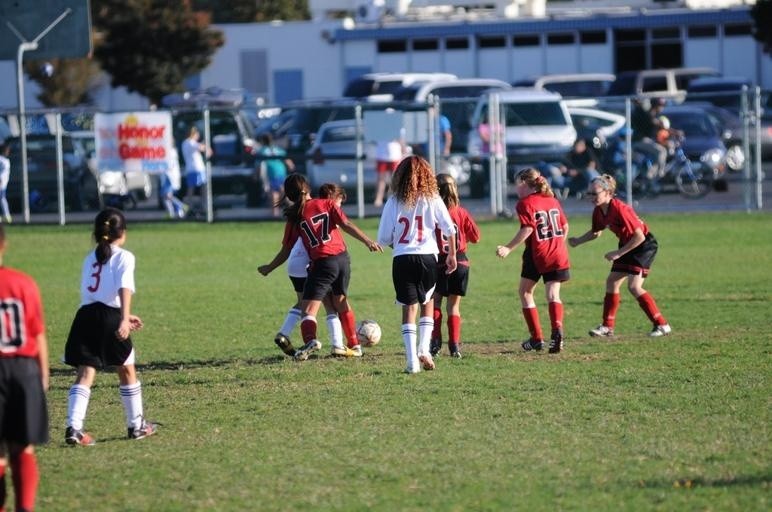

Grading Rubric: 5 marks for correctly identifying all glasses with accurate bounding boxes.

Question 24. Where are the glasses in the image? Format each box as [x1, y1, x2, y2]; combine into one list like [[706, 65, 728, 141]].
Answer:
[[586, 190, 605, 196]]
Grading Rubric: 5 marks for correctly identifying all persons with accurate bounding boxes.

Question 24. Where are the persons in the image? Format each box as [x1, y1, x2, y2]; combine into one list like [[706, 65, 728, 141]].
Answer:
[[427, 105, 452, 175], [428, 173, 481, 359], [494, 166, 570, 355], [370, 107, 414, 208], [627, 97, 684, 198], [0, 219, 53, 511], [272, 180, 356, 357], [87, 125, 213, 222], [377, 154, 458, 374], [559, 136, 598, 201], [258, 174, 385, 363], [567, 171, 673, 339], [479, 111, 509, 178], [1, 142, 13, 224], [57, 207, 164, 446], [256, 132, 297, 219]]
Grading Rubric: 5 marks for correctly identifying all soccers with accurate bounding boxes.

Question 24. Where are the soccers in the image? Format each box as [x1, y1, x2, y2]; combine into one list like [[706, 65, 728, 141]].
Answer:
[[356, 320, 381, 346]]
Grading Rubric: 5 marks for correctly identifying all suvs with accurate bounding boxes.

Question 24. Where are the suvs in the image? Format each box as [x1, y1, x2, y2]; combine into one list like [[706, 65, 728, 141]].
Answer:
[[4, 131, 156, 214], [162, 85, 269, 208]]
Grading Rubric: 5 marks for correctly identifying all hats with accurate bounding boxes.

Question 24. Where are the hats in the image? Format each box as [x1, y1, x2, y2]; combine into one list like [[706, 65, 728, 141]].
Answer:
[[649, 97, 668, 106]]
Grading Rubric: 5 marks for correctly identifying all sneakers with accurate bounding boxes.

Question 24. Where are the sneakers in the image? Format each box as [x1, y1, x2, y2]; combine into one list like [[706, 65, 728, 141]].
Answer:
[[647, 323, 672, 338], [588, 324, 616, 339], [521, 337, 547, 354], [274, 330, 462, 375], [545, 333, 565, 355], [127, 419, 157, 440], [64, 426, 96, 446]]
